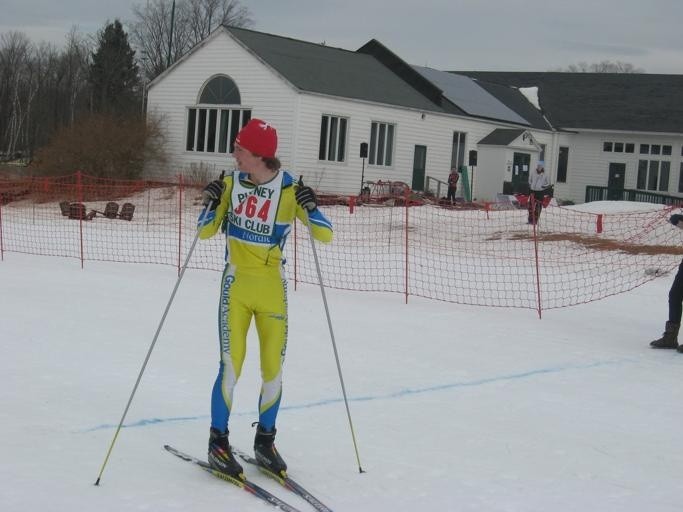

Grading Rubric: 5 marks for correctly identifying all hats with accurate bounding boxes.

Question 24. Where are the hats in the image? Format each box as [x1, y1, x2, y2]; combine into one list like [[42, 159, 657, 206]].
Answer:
[[234, 118, 277, 159]]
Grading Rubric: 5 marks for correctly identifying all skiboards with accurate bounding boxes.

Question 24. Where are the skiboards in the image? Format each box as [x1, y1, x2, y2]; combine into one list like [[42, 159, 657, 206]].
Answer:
[[164, 443, 332, 512]]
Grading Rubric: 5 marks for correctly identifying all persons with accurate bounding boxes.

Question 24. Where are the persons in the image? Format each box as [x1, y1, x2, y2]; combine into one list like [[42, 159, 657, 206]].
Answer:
[[525, 159, 550, 226], [195, 117, 333, 479], [647, 214, 683, 354], [446, 165, 459, 206]]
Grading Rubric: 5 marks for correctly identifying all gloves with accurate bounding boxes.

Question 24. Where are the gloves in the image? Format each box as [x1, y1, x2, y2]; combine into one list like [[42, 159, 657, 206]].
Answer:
[[293, 185, 318, 212], [202, 179, 226, 211]]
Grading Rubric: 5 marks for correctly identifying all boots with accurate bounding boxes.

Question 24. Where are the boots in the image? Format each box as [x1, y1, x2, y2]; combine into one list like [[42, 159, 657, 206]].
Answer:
[[252, 421, 287, 474], [650, 321, 683, 354], [207, 426, 243, 475]]
